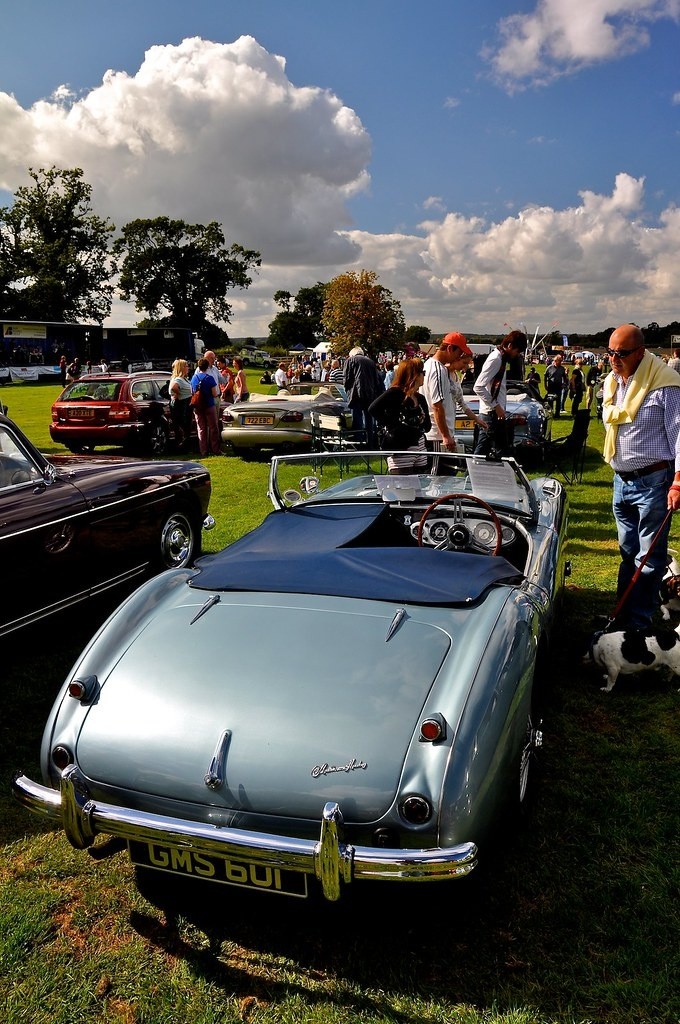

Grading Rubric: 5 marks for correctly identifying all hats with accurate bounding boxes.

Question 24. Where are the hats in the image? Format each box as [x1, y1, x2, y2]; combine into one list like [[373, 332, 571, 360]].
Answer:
[[443, 332, 472, 354], [531, 368, 535, 371]]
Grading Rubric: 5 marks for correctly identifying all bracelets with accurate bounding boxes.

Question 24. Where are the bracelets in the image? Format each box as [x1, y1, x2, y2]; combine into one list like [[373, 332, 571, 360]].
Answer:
[[669, 485, 680, 491], [673, 481, 680, 485]]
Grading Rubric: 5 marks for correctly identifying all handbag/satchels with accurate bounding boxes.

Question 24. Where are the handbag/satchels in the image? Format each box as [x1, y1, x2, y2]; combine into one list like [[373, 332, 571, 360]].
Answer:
[[378, 387, 411, 439]]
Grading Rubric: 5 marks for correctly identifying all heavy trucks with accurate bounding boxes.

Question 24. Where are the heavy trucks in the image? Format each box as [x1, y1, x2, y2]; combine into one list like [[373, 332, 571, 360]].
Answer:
[[101, 326, 205, 370]]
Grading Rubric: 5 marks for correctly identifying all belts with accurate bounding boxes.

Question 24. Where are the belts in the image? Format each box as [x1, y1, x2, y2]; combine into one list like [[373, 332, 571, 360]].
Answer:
[[616, 457, 675, 481]]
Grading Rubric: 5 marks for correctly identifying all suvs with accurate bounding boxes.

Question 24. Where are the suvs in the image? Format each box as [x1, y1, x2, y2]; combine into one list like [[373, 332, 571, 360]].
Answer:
[[49, 372, 230, 458]]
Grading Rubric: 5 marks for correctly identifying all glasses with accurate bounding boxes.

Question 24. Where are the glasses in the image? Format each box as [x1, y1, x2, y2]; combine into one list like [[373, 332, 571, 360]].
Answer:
[[606, 347, 642, 358], [419, 371, 425, 376]]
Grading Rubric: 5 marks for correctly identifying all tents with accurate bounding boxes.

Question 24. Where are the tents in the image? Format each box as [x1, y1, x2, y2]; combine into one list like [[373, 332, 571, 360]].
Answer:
[[289, 343, 306, 351]]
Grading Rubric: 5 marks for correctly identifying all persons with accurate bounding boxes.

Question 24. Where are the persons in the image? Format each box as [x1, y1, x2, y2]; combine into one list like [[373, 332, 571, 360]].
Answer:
[[168, 331, 680, 475], [60, 356, 129, 389], [593, 325, 680, 633]]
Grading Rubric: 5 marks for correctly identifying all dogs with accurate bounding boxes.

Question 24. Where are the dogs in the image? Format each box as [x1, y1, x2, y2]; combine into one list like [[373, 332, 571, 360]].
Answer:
[[656, 545, 680, 621], [583, 624, 680, 692]]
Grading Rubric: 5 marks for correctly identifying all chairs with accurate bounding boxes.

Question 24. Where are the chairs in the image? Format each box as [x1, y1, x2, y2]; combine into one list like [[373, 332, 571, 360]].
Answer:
[[310, 405, 370, 482], [541, 409, 591, 484], [277, 389, 291, 396], [462, 388, 476, 395], [97, 386, 109, 399], [507, 389, 522, 395], [319, 387, 332, 396]]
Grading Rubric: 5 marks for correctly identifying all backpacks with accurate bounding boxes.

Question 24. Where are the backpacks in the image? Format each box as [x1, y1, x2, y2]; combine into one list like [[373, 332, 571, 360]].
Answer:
[[188, 374, 210, 409]]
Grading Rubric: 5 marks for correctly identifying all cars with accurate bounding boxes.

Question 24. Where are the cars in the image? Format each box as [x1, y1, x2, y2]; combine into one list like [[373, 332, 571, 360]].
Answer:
[[13, 445, 569, 902], [421, 377, 557, 459], [0, 408, 214, 656], [221, 380, 353, 458]]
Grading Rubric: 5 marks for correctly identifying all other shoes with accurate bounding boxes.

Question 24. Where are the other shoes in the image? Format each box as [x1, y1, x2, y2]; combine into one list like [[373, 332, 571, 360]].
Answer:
[[212, 451, 226, 456], [201, 453, 208, 457], [592, 626, 617, 638]]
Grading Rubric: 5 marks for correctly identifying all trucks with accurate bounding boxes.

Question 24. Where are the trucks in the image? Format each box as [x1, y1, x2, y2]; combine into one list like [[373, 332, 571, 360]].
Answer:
[[243, 351, 278, 368], [467, 344, 495, 354]]
